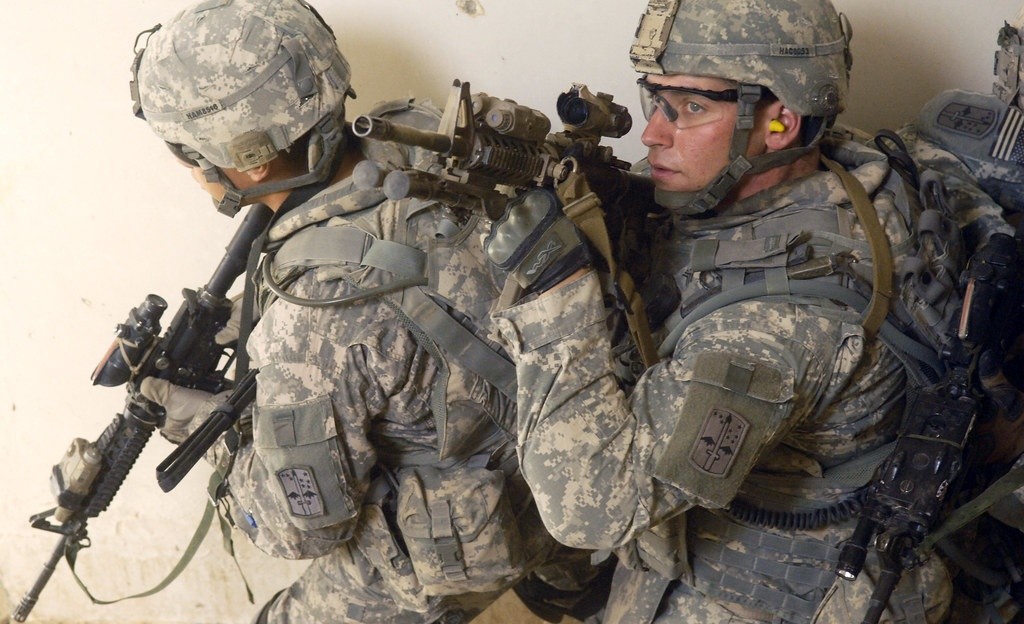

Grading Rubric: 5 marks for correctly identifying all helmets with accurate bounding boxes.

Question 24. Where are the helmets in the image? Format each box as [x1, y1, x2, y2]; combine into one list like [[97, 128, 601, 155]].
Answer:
[[129, 0, 352, 171], [630, 0, 851, 116]]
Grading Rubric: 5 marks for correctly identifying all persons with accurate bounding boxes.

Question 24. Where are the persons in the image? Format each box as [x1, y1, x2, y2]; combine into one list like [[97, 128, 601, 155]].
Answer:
[[477, 1, 919, 624], [128, 0, 566, 624]]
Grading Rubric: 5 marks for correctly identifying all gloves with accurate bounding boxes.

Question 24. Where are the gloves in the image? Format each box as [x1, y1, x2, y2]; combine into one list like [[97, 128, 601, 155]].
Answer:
[[140, 378, 215, 447], [213, 288, 262, 346], [480, 186, 592, 293]]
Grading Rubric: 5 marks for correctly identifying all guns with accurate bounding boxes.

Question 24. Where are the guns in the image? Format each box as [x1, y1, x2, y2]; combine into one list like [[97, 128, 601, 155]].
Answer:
[[352, 84, 670, 233], [12, 204, 272, 623]]
